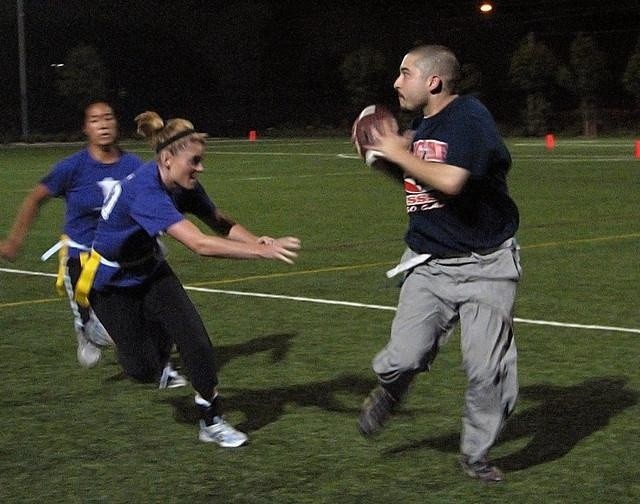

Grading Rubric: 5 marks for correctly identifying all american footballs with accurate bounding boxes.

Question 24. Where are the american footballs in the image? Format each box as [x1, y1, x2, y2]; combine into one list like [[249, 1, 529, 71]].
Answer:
[[352, 104, 399, 168]]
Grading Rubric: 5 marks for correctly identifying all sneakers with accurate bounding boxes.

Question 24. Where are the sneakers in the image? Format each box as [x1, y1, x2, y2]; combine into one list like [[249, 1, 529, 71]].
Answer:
[[358, 387, 397, 437], [76, 329, 101, 369], [198, 417, 248, 448], [159, 363, 188, 391], [461, 456, 504, 483]]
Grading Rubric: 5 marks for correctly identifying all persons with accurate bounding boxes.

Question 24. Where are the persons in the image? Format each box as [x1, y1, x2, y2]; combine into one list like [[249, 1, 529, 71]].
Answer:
[[83, 111, 301, 448], [0, 96, 189, 391], [353, 42, 524, 479]]
